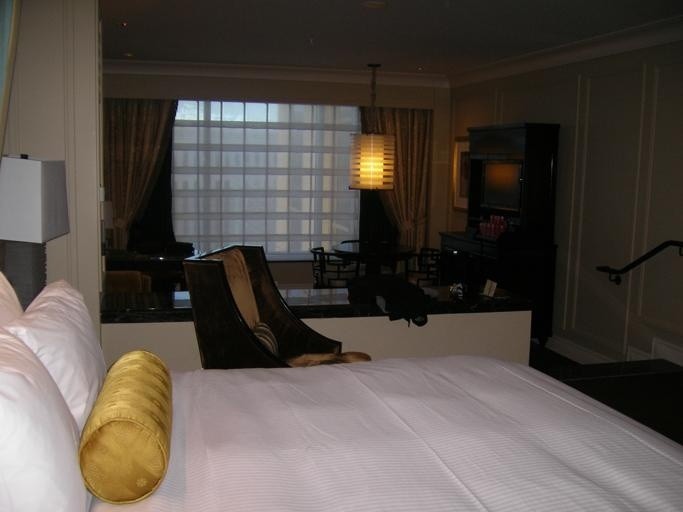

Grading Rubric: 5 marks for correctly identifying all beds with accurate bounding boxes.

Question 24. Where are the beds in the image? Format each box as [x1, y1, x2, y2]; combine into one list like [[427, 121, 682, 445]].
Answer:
[[0, 267, 682, 512]]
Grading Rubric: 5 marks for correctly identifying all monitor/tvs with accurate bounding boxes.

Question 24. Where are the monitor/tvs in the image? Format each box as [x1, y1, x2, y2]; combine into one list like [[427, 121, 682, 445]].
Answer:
[[478, 159, 523, 213]]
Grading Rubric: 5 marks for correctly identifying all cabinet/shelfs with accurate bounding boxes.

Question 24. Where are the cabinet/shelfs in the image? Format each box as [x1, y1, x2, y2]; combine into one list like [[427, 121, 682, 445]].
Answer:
[[439, 123, 570, 340]]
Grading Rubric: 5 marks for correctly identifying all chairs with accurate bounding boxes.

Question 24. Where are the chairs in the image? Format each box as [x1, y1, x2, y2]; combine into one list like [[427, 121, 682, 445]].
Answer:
[[102, 240, 196, 322], [180, 242, 373, 370], [306, 240, 438, 291]]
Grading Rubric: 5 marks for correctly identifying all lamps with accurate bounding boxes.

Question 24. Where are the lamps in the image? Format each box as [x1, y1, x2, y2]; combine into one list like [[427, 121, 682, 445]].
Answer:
[[0, 153, 71, 312], [349, 62, 402, 192]]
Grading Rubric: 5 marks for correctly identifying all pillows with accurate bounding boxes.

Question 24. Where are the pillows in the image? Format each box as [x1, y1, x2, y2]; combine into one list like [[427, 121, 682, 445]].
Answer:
[[5, 279, 109, 434], [2, 328, 96, 512], [1, 272, 26, 331], [78, 350, 173, 508]]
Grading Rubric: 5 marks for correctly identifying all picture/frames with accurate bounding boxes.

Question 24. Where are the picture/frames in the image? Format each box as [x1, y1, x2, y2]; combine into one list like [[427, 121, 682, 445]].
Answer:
[[449, 136, 471, 212]]
